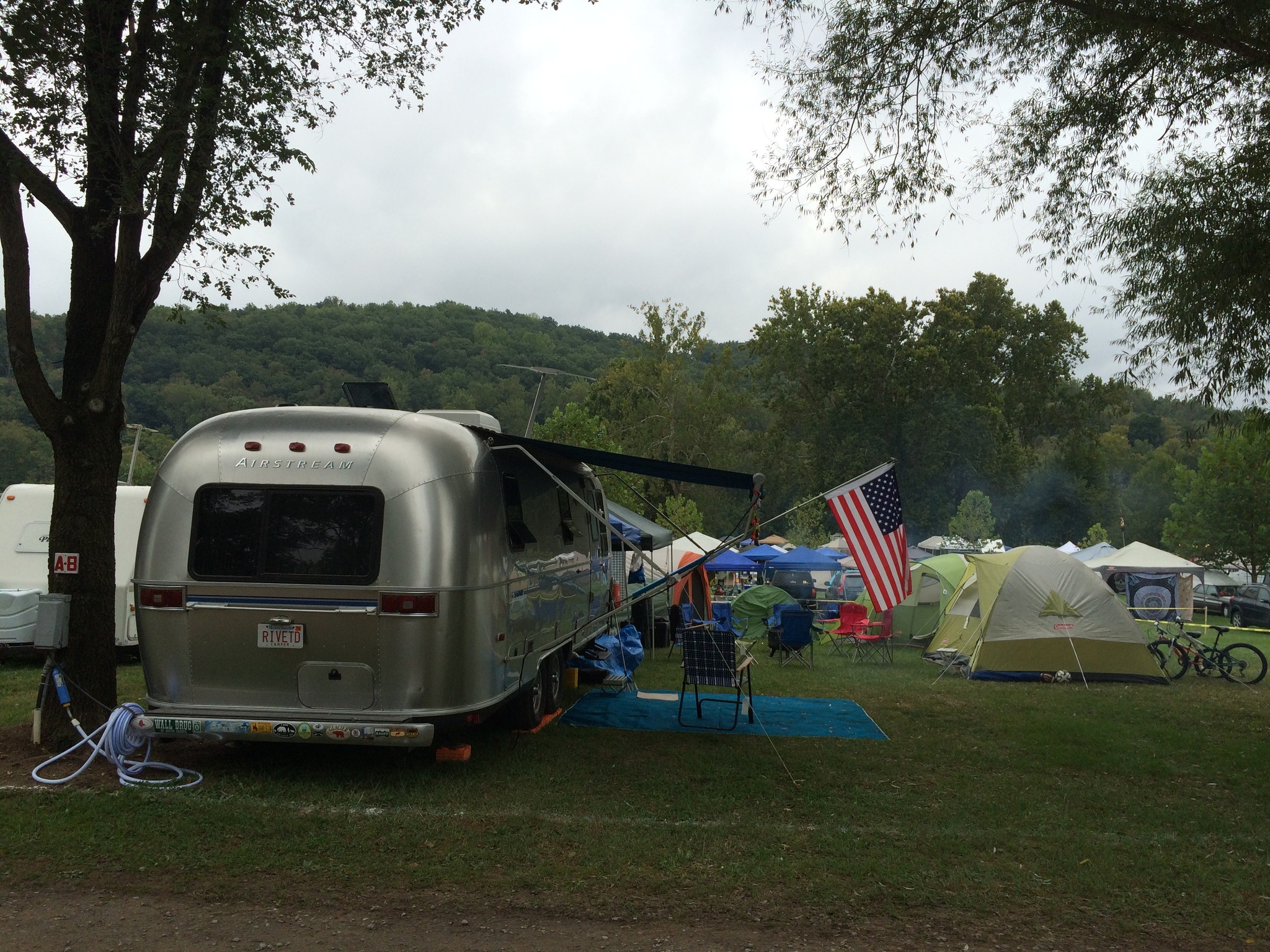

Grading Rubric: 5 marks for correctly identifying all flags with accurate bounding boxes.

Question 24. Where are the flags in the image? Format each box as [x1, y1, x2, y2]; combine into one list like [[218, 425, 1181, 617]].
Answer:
[[822, 462, 913, 613]]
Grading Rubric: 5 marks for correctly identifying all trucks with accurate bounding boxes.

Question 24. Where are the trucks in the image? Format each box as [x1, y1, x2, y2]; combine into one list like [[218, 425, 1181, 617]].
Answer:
[[129, 379, 622, 753]]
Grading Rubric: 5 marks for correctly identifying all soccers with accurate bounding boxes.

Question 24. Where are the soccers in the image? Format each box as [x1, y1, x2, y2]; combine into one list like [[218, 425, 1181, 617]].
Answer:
[[1056, 669, 1072, 685]]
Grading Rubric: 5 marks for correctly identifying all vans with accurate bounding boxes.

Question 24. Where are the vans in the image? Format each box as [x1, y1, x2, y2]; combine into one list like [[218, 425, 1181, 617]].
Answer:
[[0, 480, 151, 664]]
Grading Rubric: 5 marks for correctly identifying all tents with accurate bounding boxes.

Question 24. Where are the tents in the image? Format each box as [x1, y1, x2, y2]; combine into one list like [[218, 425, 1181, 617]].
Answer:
[[604, 497, 1238, 692]]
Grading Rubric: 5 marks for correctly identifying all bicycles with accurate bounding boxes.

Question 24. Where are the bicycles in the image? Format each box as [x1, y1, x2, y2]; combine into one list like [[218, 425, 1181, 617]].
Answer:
[[1146, 613, 1268, 685]]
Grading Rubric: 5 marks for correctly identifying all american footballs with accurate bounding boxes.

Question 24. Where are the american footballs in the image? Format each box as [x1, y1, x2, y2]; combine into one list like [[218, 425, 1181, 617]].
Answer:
[[1040, 673, 1056, 683]]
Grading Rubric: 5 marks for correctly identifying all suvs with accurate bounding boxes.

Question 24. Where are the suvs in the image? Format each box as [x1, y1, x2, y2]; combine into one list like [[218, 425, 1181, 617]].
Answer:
[[765, 570, 817, 610]]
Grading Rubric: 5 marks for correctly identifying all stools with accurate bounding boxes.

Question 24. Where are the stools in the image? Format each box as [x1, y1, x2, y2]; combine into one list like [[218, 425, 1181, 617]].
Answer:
[[734, 636, 759, 666], [935, 647, 959, 673]]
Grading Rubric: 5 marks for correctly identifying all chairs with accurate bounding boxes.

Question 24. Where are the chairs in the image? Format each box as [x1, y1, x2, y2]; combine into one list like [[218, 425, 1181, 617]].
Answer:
[[664, 601, 761, 660], [677, 628, 755, 732], [761, 602, 814, 670], [711, 579, 725, 593], [816, 601, 895, 665]]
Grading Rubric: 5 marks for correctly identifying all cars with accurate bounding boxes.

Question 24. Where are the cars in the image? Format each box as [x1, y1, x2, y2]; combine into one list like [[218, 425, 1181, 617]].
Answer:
[[1229, 582, 1270, 630], [824, 567, 866, 601], [1193, 584, 1239, 618]]
